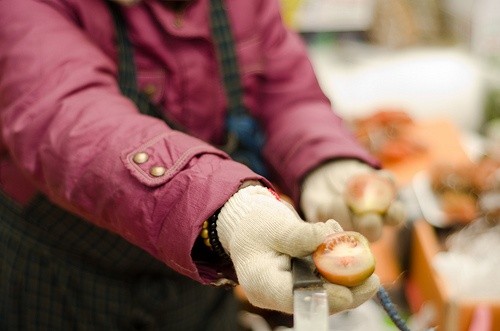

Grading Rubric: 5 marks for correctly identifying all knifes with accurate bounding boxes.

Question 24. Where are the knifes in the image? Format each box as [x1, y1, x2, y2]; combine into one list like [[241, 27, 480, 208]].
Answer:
[[292, 258, 329, 331]]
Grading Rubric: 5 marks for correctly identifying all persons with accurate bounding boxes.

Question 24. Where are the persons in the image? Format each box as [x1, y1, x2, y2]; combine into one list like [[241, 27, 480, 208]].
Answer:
[[0, 0, 405, 331]]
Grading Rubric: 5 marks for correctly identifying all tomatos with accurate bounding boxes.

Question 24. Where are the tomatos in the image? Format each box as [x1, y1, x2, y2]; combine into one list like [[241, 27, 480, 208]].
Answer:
[[313, 172, 398, 290]]
